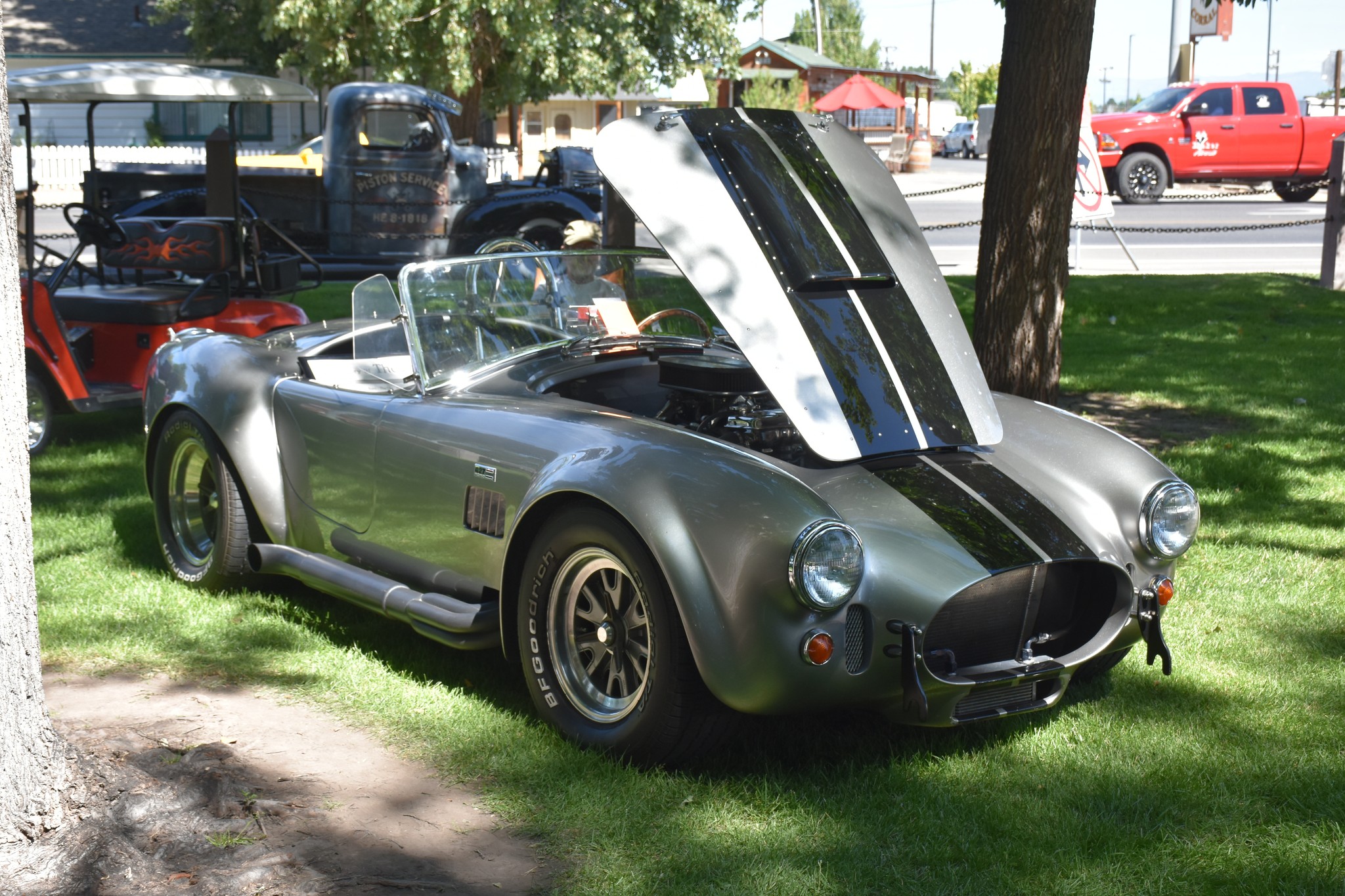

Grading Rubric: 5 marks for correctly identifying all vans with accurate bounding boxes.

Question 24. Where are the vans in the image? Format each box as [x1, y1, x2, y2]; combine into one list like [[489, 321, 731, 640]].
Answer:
[[939, 120, 982, 159]]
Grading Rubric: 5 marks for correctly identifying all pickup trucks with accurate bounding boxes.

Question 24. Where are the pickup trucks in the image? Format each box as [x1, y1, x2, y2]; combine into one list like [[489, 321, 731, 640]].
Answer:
[[82, 81, 606, 285], [1093, 80, 1345, 205]]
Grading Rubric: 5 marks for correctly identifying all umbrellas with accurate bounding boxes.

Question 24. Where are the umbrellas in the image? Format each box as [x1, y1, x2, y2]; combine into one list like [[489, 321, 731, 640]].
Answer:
[[812, 74, 905, 134]]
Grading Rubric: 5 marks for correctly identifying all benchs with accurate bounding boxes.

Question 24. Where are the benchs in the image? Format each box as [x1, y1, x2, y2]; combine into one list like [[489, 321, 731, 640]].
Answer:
[[53, 217, 231, 326]]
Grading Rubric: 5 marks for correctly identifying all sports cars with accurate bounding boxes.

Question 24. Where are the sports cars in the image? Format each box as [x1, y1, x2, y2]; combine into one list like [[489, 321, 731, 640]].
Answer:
[[143, 106, 1199, 767]]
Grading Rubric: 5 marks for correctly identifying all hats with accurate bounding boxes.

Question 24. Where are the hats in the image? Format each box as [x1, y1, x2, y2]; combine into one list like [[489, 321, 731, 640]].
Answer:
[[562, 220, 603, 247]]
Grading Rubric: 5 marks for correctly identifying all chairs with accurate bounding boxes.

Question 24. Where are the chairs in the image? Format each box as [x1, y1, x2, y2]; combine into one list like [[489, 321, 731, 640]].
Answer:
[[877, 133, 917, 175]]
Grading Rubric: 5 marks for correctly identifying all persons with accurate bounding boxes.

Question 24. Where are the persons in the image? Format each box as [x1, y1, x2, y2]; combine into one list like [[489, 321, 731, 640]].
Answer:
[[528, 220, 643, 355]]
